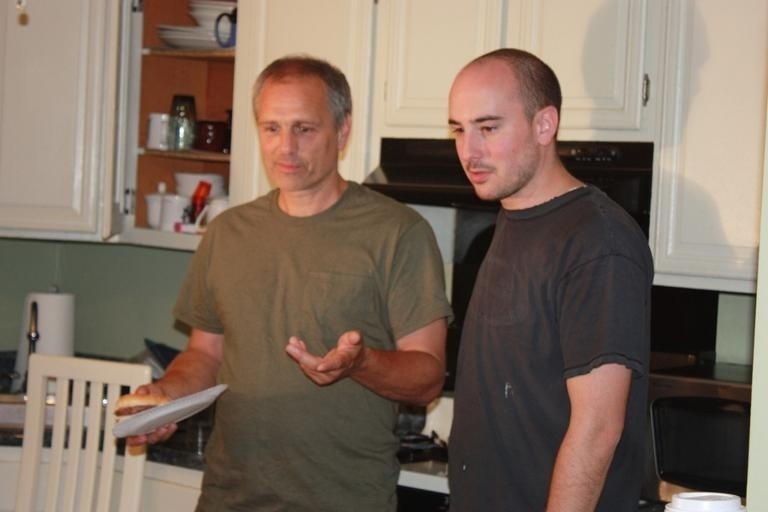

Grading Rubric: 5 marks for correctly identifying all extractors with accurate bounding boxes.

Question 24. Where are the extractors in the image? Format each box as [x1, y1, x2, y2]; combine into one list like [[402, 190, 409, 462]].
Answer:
[[361, 140, 653, 215]]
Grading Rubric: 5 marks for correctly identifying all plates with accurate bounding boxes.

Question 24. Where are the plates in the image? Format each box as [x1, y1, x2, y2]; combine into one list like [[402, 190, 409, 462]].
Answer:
[[154, 0, 237, 52], [110, 381, 234, 441], [142, 171, 229, 236]]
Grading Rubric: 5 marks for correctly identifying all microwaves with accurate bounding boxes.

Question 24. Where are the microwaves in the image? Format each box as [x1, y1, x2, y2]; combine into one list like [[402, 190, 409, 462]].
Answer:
[[639, 361, 752, 507]]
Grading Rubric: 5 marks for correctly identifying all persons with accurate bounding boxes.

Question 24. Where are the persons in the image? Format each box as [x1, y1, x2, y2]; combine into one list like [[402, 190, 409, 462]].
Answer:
[[438, 47, 661, 512], [124, 54, 454, 512]]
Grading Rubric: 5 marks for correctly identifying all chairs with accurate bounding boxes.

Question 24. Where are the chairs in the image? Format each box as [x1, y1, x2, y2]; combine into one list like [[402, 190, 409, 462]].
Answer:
[[14, 353, 153, 512]]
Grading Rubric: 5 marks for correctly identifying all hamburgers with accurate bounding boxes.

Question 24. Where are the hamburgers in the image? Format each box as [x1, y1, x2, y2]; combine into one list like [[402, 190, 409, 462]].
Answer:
[[111, 392, 174, 427]]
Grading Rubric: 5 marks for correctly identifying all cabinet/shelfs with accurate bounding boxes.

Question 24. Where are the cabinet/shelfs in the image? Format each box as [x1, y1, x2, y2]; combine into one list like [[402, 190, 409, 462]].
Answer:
[[650, 0, 768, 294], [118, 0, 370, 250], [3, 1, 115, 241], [373, 0, 657, 143]]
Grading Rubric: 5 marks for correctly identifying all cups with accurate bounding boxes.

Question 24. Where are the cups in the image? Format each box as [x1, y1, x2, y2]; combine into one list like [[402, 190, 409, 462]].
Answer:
[[148, 91, 233, 153]]
